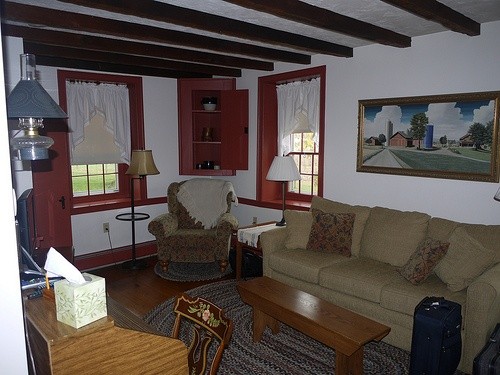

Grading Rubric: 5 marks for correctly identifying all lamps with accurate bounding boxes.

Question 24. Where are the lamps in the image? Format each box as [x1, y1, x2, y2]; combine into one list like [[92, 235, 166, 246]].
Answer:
[[266, 155, 302, 225], [8, 53, 71, 160], [115, 149, 160, 265]]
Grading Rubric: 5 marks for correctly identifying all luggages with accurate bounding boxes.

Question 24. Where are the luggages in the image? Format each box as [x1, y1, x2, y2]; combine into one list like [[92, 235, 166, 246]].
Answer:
[[473, 323, 500, 375], [408, 296, 462, 375]]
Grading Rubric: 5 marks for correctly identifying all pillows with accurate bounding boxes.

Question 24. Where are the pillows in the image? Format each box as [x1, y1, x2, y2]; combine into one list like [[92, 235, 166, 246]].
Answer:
[[283, 209, 313, 249], [434, 226, 499, 292], [305, 209, 356, 258], [396, 237, 450, 286]]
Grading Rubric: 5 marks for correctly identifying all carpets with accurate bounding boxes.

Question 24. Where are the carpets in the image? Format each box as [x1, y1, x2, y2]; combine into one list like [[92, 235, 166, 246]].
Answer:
[[142, 278, 411, 375], [154, 262, 233, 282]]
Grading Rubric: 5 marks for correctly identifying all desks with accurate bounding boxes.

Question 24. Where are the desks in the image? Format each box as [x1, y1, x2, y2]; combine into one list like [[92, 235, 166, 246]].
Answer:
[[21, 287, 189, 375]]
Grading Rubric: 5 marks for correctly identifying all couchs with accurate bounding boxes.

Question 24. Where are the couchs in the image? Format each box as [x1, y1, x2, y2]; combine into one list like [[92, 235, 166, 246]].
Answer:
[[147, 177, 240, 264], [255, 196, 499, 375]]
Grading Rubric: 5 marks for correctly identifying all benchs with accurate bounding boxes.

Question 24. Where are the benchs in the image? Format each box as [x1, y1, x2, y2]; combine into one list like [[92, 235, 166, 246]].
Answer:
[[237, 275, 392, 375]]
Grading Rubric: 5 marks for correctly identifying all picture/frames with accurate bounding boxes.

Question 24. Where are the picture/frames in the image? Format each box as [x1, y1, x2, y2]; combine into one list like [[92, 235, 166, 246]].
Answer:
[[356, 89, 500, 183]]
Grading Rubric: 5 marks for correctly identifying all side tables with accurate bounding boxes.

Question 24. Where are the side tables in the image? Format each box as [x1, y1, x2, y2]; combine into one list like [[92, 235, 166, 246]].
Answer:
[[236, 222, 289, 276]]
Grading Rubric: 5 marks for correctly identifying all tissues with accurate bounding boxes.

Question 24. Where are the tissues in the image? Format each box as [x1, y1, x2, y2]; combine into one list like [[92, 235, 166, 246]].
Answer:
[[43, 247, 108, 331]]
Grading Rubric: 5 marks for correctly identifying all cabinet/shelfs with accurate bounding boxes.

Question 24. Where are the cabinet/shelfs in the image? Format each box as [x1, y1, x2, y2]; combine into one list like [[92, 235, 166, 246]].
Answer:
[[176, 78, 249, 176]]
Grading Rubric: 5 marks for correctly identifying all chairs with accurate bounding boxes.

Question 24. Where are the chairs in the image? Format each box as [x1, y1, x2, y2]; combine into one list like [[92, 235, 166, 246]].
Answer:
[[169, 293, 233, 375]]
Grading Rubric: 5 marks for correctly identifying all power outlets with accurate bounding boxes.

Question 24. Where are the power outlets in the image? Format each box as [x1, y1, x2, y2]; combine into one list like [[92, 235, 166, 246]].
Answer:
[[102, 221, 110, 233], [251, 216, 257, 224]]
